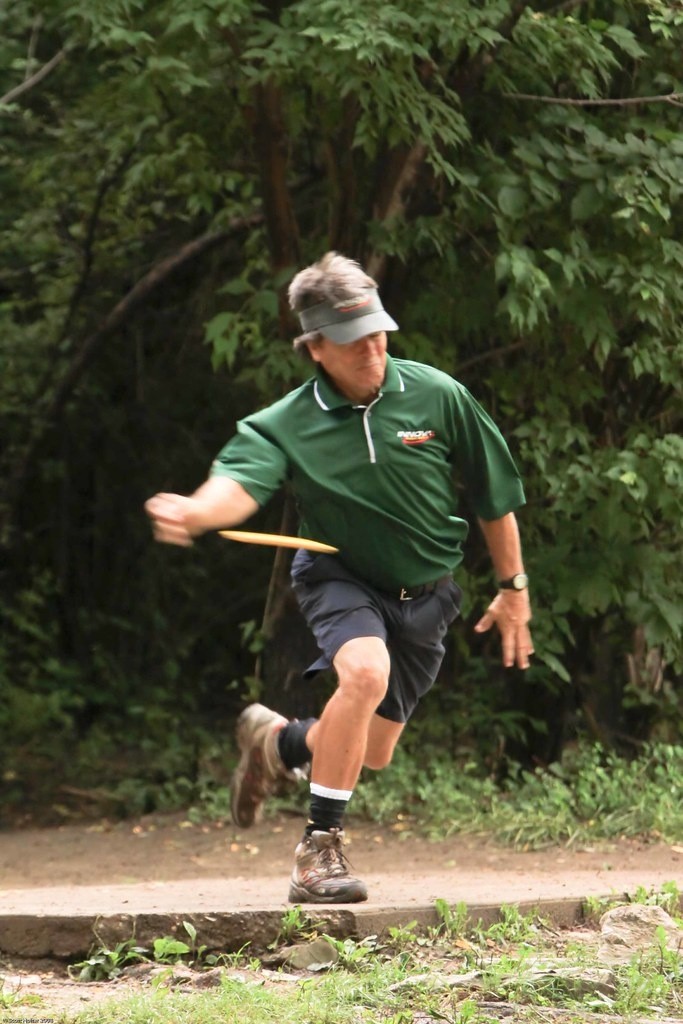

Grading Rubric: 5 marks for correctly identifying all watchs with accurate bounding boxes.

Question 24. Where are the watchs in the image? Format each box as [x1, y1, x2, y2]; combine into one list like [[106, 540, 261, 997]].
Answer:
[[497, 573, 529, 591]]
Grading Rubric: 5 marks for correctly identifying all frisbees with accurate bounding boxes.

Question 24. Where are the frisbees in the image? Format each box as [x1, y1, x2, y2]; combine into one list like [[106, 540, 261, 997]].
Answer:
[[216, 529, 340, 555]]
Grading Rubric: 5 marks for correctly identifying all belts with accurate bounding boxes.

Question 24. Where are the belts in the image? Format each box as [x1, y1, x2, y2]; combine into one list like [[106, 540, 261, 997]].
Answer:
[[382, 574, 452, 601]]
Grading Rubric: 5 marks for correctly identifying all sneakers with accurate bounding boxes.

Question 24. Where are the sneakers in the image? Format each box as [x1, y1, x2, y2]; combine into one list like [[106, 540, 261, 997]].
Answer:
[[228, 702, 296, 828], [289, 828, 367, 905]]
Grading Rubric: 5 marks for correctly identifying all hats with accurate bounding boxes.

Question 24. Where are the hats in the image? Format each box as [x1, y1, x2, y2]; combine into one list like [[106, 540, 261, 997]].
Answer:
[[299, 286, 401, 344]]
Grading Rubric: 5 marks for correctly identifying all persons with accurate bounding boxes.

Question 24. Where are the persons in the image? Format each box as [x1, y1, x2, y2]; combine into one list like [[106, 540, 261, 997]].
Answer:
[[146, 256, 533, 904]]
